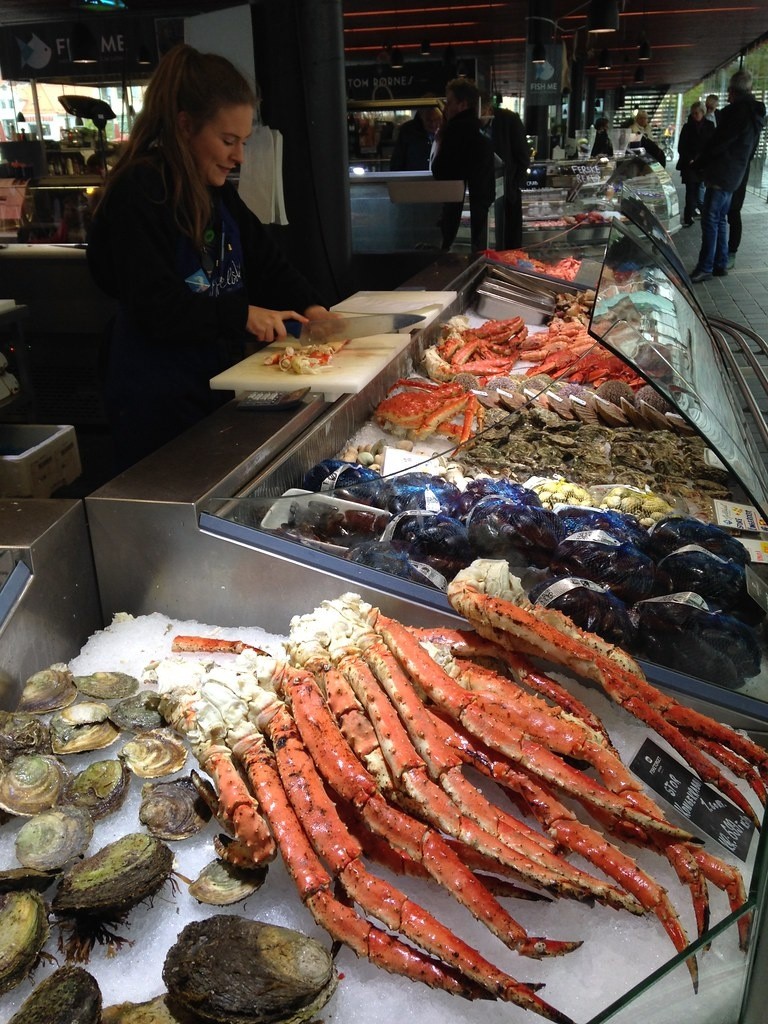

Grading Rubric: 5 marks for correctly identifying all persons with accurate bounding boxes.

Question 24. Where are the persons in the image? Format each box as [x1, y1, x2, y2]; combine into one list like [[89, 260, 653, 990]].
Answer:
[[430, 80, 495, 252], [86, 45, 344, 477], [676, 101, 714, 226], [689, 71, 766, 282], [629, 111, 666, 167], [480, 101, 529, 250], [698, 94, 720, 205], [591, 118, 613, 158], [390, 94, 442, 170]]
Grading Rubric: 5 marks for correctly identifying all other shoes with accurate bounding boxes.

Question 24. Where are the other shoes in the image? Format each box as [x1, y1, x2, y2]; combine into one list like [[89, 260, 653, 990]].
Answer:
[[712, 266, 728, 276], [726, 254, 735, 269], [684, 219, 694, 228], [690, 269, 713, 281]]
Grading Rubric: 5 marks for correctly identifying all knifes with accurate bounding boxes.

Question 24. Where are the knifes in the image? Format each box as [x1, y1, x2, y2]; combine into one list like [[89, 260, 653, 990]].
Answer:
[[280, 312, 427, 347]]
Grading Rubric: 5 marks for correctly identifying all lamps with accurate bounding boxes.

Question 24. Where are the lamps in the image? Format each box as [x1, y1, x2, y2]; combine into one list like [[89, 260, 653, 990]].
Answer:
[[637, 0, 650, 60], [390, 0, 404, 69], [137, 44, 152, 65], [586, 0, 620, 34], [596, 34, 612, 70], [68, 0, 98, 64], [419, 0, 430, 56], [634, 65, 644, 84]]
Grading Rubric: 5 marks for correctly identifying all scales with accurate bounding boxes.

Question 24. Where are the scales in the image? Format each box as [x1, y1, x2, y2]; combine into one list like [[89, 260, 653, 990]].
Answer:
[[31, 94, 116, 186]]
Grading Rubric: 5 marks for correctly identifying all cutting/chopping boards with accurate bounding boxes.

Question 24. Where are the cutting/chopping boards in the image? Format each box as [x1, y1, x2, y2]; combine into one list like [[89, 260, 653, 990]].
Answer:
[[209, 291, 457, 394]]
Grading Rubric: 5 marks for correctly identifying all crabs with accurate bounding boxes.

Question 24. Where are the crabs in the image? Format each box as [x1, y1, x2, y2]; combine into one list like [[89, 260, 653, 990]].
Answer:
[[172, 208, 768, 1024]]
